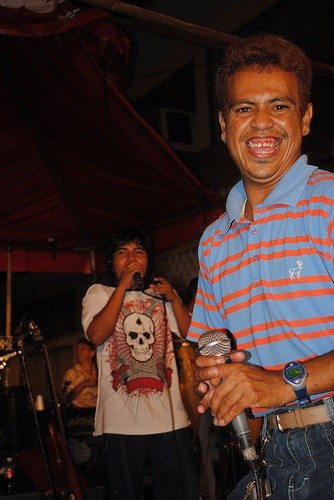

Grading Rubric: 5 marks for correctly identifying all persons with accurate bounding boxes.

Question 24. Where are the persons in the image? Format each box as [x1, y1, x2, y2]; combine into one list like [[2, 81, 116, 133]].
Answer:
[[81, 229, 198, 500], [186, 38, 333, 500], [61, 339, 98, 418], [185, 277, 233, 500]]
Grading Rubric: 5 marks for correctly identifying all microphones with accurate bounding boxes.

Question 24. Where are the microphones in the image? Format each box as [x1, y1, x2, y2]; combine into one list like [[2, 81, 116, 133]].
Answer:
[[134, 272, 144, 290], [197, 329, 258, 474]]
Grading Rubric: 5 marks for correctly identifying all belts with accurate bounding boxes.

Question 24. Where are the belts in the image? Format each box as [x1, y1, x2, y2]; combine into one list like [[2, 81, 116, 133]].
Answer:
[[267, 403, 330, 431]]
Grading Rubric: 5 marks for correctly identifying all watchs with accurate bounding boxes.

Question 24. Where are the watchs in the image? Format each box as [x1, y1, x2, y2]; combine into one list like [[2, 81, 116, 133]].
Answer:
[[283, 361, 311, 406]]
